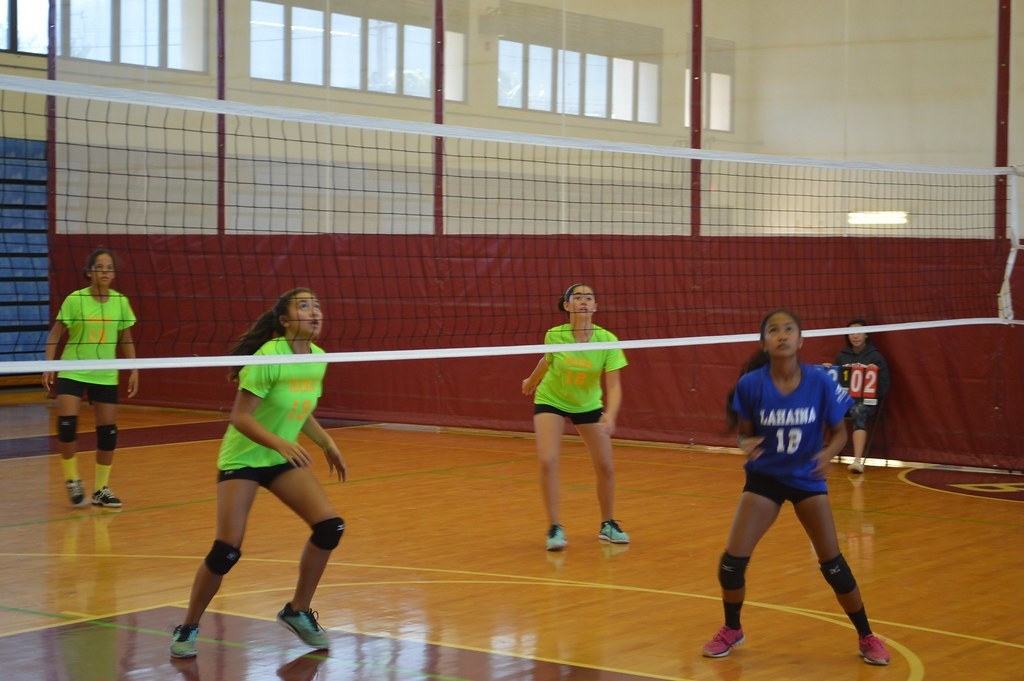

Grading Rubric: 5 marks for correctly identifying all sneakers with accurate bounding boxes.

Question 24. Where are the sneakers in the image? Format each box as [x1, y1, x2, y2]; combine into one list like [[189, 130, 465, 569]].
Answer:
[[276, 602, 331, 649], [91, 485, 122, 507], [169, 624, 199, 658], [598, 518, 631, 543], [545, 523, 566, 550], [858, 634, 890, 665], [702, 625, 745, 657], [65, 479, 85, 505]]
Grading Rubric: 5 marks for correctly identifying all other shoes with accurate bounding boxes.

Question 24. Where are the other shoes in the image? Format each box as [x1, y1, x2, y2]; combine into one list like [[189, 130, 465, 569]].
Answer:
[[847, 457, 864, 474]]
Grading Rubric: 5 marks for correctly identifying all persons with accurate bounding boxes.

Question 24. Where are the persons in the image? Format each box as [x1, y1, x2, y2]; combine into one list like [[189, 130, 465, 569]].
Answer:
[[170, 289, 347, 661], [42, 248, 138, 508], [522, 283, 629, 552], [701, 309, 890, 666], [822, 319, 889, 473]]
